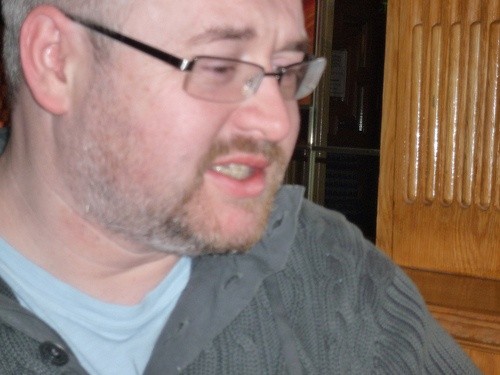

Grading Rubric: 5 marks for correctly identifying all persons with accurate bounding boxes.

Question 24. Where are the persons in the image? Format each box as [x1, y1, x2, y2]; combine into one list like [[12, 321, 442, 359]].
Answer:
[[0, 0, 484, 375]]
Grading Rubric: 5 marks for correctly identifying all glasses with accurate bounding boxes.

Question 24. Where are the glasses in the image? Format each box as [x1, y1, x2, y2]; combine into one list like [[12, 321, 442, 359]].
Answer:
[[66, 12, 328, 104]]
